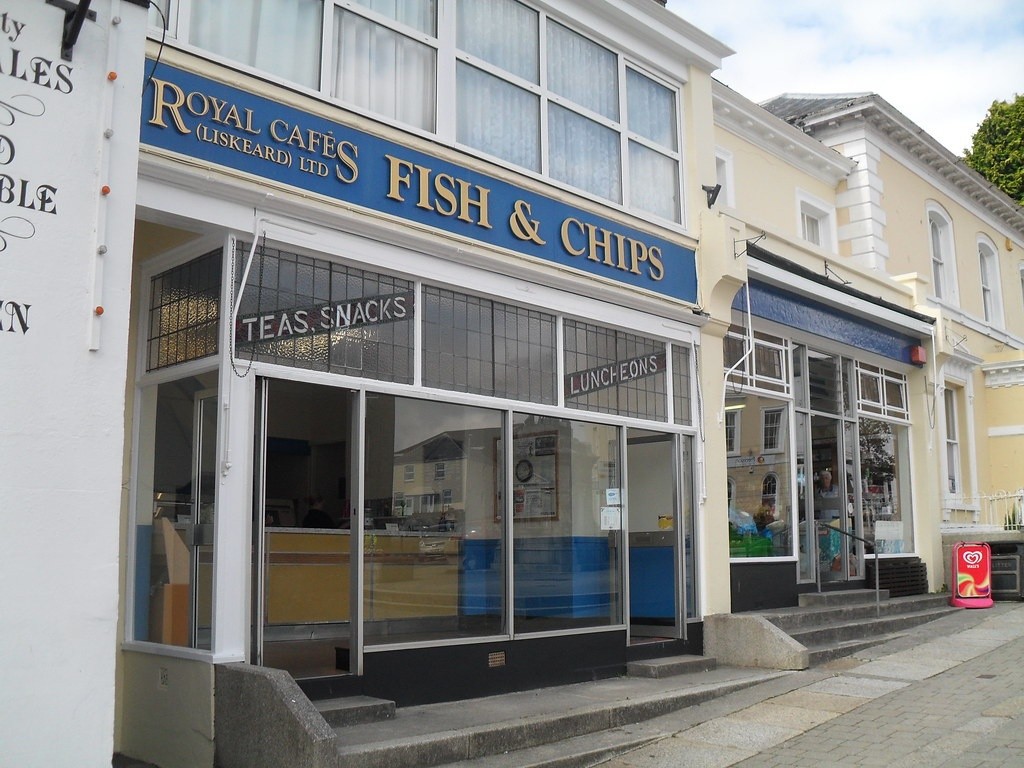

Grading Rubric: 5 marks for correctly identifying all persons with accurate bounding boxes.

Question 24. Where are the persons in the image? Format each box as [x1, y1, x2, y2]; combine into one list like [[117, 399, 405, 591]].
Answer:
[[302, 492, 336, 529], [814, 471, 850, 519]]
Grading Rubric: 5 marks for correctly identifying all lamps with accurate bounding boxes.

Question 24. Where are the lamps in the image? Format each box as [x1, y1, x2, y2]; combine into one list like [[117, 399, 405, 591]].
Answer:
[[46, 0, 97, 61], [702, 183, 722, 210]]
